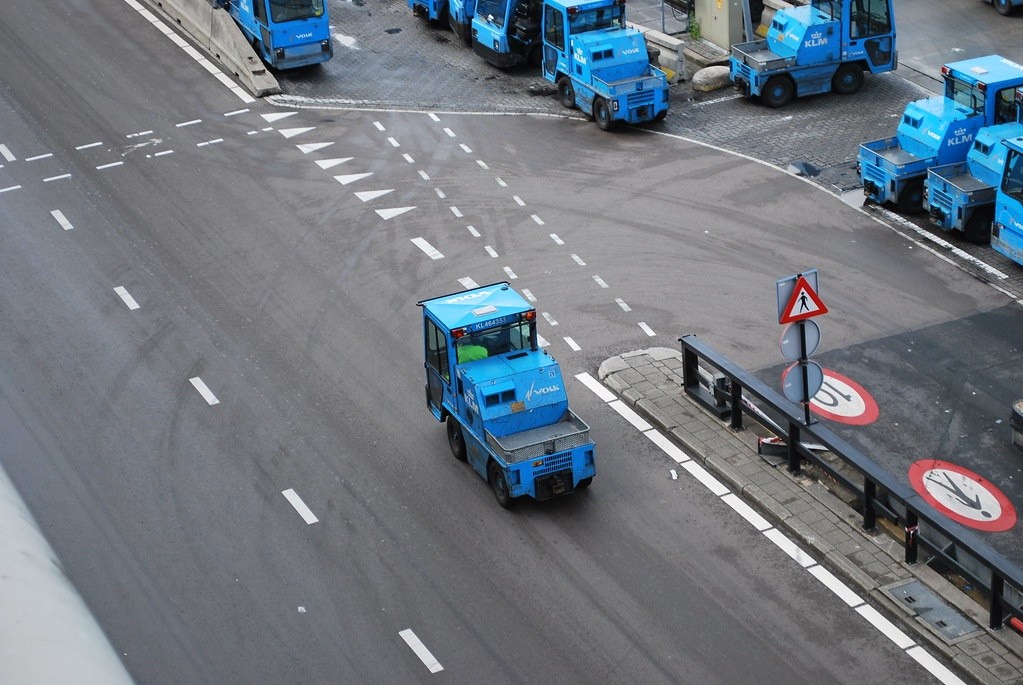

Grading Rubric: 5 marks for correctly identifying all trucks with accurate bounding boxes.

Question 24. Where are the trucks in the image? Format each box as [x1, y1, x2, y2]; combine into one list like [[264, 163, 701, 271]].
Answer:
[[226, 0, 333, 71], [415, 279, 598, 509], [853, 55, 1023, 267], [728, 0, 897, 108], [541, 1, 670, 130], [406, 0, 543, 75], [983, 0, 1023, 17]]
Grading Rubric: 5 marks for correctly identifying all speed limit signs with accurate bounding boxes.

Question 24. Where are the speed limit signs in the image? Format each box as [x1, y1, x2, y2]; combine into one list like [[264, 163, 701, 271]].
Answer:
[[808, 367, 879, 426]]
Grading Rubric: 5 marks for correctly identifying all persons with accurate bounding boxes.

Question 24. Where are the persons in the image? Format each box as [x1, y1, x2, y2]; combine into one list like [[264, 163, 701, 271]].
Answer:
[[445, 340, 488, 380]]
